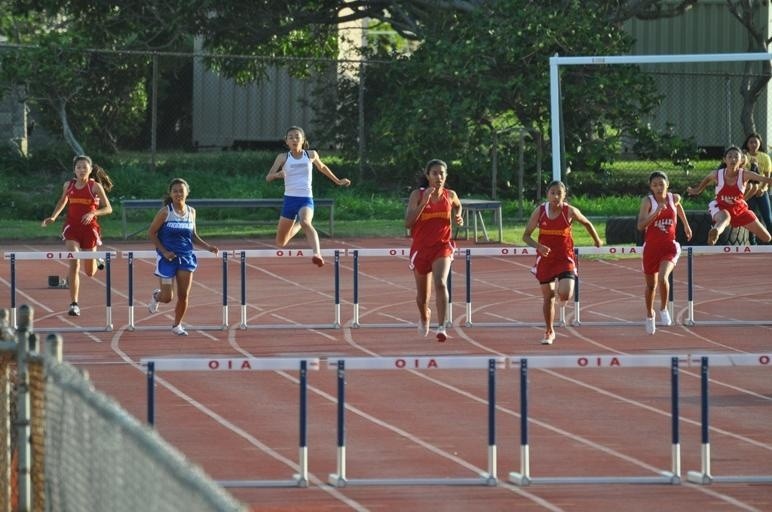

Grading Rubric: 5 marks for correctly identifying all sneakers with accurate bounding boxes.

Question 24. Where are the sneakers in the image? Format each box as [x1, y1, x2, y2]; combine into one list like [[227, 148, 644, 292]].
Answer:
[[417, 307, 432, 337], [67, 302, 81, 317], [657, 308, 672, 326], [706, 226, 720, 245], [95, 256, 105, 271], [311, 254, 325, 267], [147, 287, 161, 313], [541, 330, 556, 345], [436, 327, 446, 343], [169, 323, 189, 338], [644, 310, 655, 335]]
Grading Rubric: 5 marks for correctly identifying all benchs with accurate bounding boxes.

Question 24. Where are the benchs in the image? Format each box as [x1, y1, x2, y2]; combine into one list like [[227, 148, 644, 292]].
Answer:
[[402, 196, 506, 247], [114, 196, 338, 247]]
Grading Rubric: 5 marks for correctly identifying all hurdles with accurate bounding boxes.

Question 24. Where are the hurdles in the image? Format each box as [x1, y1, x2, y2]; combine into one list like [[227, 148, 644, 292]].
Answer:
[[508, 353, 690, 485], [681, 245, 772, 326], [459, 247, 567, 327], [572, 247, 676, 327], [122, 250, 233, 332], [326, 355, 507, 489], [686, 353, 771, 484], [347, 248, 459, 328], [4, 250, 118, 332], [139, 357, 321, 488], [235, 248, 346, 331]]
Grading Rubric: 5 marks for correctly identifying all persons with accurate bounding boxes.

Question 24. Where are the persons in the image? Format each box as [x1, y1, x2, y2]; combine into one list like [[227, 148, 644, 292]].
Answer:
[[638, 172, 692, 335], [43, 155, 113, 317], [149, 178, 219, 336], [266, 127, 351, 267], [406, 159, 464, 343], [687, 133, 772, 245], [523, 180, 603, 344]]
